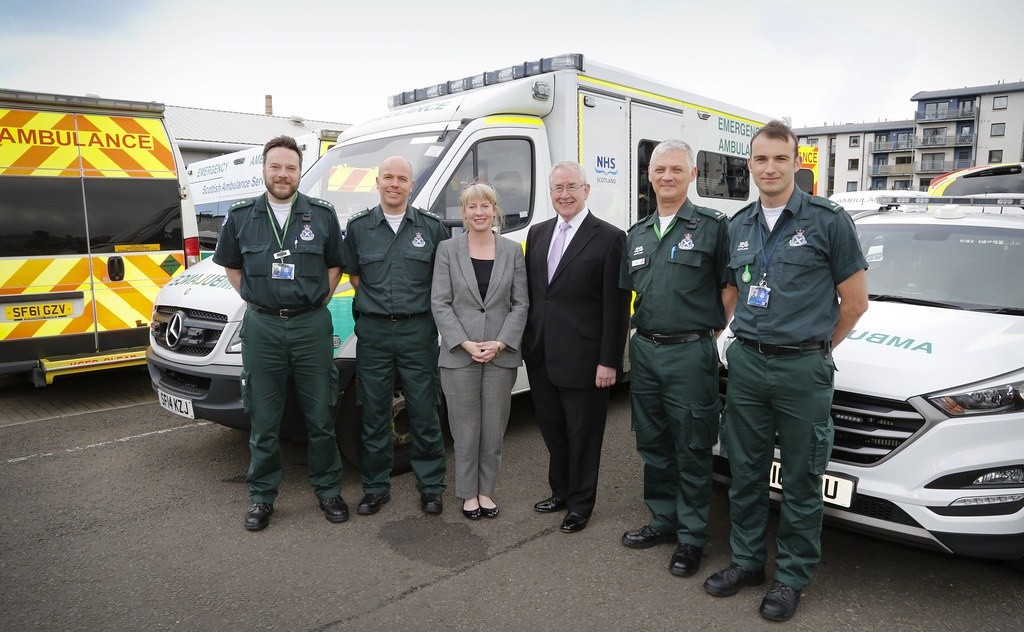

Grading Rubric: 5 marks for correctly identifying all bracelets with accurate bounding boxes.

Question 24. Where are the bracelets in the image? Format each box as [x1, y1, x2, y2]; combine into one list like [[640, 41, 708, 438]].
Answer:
[[496, 341, 500, 352]]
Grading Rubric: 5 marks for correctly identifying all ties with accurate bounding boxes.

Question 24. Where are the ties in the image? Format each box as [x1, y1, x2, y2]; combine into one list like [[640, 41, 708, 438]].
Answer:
[[548, 221, 571, 285]]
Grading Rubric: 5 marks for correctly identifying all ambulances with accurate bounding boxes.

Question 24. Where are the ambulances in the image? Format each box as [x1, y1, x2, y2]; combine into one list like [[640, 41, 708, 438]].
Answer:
[[146, 53, 819, 478], [713, 190, 1024, 561], [0, 87, 201, 387]]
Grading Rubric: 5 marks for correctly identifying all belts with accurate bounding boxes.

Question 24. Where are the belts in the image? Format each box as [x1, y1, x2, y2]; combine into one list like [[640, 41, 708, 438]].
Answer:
[[737, 335, 833, 357], [637, 328, 712, 345], [354, 310, 430, 323], [248, 299, 323, 319]]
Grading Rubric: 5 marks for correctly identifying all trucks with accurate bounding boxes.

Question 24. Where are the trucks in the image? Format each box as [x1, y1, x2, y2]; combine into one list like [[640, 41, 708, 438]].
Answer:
[[186, 133, 379, 261]]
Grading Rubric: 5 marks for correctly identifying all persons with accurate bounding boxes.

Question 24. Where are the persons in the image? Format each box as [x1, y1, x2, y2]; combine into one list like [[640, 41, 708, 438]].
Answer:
[[520, 161, 632, 533], [430, 184, 529, 520], [340, 155, 450, 516], [212, 137, 350, 532], [617, 136, 738, 579], [703, 120, 869, 622]]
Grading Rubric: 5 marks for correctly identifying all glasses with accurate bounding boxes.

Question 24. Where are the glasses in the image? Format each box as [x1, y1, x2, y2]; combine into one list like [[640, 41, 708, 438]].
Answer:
[[549, 184, 586, 193]]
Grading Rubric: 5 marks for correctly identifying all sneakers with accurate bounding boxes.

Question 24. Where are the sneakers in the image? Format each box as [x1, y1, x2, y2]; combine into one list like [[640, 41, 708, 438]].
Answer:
[[421, 492, 443, 514], [670, 543, 703, 578], [319, 495, 350, 523], [704, 560, 766, 598], [356, 490, 390, 516], [621, 523, 677, 550]]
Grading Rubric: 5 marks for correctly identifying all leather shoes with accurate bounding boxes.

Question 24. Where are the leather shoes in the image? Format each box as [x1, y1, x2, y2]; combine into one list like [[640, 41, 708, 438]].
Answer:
[[759, 580, 800, 622], [533, 495, 564, 514], [560, 511, 591, 533], [462, 499, 481, 520], [480, 495, 499, 520], [245, 501, 274, 532]]
